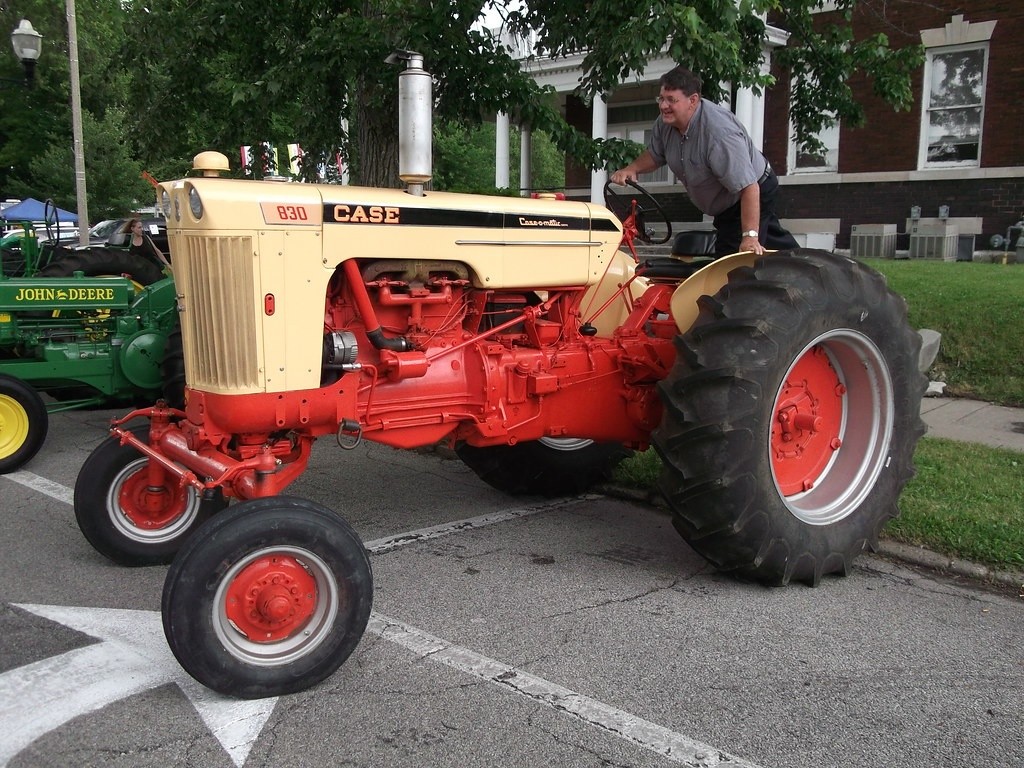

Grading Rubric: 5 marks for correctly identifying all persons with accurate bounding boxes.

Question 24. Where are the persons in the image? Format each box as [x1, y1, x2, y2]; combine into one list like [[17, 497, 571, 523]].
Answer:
[[122, 218, 172, 271], [610, 67, 802, 262]]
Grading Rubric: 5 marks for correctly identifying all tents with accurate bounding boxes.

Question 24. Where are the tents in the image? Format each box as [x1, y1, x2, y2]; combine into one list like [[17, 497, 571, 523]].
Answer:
[[0, 198, 79, 222]]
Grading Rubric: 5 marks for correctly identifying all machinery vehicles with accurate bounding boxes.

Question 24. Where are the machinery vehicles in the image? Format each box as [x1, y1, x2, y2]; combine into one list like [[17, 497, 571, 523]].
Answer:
[[72, 46, 933, 703], [0, 196, 186, 478]]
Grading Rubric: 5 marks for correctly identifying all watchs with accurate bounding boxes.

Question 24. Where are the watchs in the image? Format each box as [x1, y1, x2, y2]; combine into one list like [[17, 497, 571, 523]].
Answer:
[[742, 229, 758, 237]]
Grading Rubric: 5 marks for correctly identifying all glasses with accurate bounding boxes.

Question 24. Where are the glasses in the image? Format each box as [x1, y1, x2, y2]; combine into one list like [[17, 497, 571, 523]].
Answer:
[[656, 96, 687, 105]]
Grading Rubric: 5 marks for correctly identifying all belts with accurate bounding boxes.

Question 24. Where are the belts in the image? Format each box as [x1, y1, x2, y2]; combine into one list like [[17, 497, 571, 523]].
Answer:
[[758, 161, 771, 184]]
